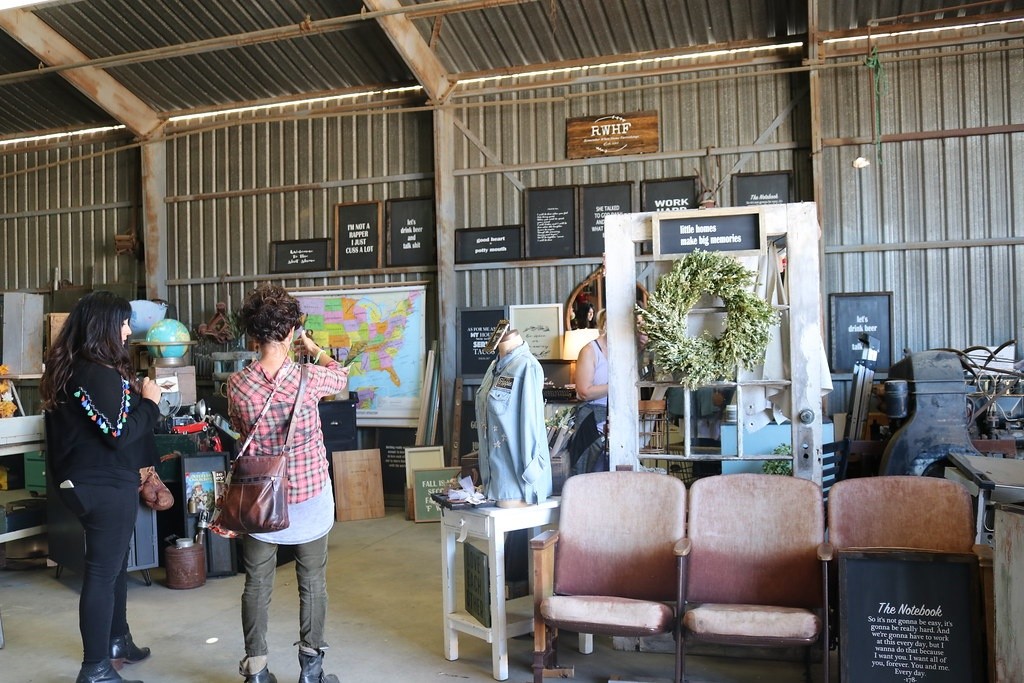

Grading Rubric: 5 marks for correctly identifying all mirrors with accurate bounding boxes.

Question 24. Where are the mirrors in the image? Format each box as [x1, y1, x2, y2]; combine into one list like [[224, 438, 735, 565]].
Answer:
[[566, 268, 653, 334]]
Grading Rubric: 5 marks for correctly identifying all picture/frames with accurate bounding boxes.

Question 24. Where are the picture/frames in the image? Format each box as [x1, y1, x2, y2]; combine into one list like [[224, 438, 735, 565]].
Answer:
[[509, 303, 564, 364], [414, 465, 465, 523], [405, 445, 445, 487], [336, 201, 383, 270]]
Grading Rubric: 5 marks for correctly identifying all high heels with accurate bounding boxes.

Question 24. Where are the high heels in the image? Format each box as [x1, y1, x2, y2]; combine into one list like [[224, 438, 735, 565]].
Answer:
[[75, 656, 145, 683], [109, 632, 151, 671]]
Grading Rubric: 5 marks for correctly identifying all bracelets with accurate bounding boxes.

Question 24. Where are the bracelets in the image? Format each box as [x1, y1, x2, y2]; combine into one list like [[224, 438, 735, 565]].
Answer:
[[314, 350, 327, 364]]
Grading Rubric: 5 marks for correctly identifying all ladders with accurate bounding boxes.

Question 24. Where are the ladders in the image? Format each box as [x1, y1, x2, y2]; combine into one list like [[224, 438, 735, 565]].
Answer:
[[841, 331, 881, 440]]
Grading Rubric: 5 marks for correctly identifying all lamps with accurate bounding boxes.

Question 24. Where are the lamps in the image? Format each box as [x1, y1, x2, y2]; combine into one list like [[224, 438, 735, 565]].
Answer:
[[564, 329, 600, 362]]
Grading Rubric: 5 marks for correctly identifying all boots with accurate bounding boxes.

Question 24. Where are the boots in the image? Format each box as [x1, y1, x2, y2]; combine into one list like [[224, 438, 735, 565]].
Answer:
[[297, 647, 339, 683], [239, 655, 277, 683]]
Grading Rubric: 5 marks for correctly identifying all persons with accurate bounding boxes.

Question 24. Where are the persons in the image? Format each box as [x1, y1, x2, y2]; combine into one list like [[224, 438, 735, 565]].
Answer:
[[474, 329, 553, 510], [226, 283, 349, 683], [570, 309, 609, 477], [37, 291, 161, 683], [576, 301, 597, 330]]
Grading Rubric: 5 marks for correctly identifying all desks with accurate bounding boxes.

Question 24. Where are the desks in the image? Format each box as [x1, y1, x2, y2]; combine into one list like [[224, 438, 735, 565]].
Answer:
[[435, 490, 595, 680]]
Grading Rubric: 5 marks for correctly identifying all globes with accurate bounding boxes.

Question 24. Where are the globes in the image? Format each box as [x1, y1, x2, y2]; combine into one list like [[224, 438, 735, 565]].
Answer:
[[146, 318, 190, 367]]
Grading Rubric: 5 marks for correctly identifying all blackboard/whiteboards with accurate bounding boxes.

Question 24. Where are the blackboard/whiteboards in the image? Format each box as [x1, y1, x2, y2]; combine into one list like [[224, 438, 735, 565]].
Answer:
[[653, 209, 768, 261], [523, 167, 793, 259], [827, 291, 894, 380], [456, 306, 509, 385], [510, 304, 564, 359], [269, 197, 524, 274], [839, 546, 998, 683]]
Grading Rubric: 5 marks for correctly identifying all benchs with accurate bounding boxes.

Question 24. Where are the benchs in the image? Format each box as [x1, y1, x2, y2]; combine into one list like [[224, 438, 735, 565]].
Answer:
[[529, 471, 988, 683]]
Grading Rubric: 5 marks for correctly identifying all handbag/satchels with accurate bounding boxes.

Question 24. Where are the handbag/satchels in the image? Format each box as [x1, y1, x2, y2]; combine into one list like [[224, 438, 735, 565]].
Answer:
[[222, 456, 290, 533]]
